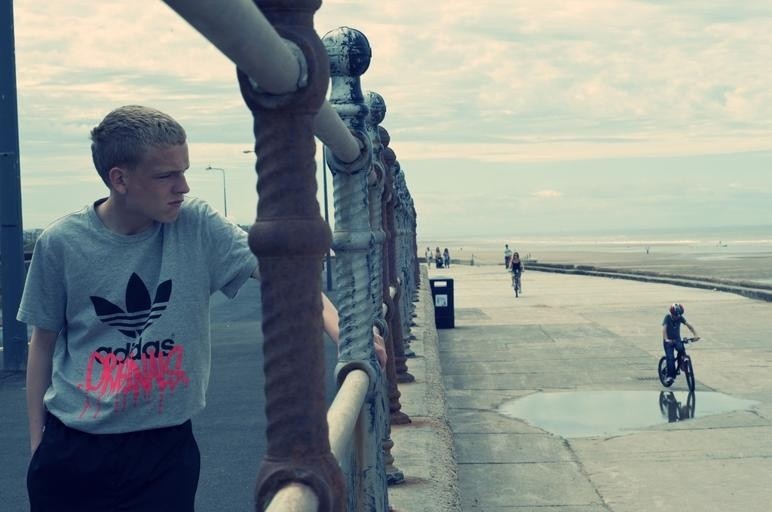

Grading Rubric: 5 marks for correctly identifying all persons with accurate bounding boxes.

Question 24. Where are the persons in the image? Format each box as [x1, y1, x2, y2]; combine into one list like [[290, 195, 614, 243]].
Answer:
[[509, 252, 524, 293], [503, 244, 511, 269], [425, 246, 450, 268], [17, 105, 387, 512], [663, 303, 698, 383]]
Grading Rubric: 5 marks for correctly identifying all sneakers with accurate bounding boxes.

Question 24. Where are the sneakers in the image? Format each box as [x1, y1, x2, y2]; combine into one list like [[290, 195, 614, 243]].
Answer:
[[666, 377, 675, 385]]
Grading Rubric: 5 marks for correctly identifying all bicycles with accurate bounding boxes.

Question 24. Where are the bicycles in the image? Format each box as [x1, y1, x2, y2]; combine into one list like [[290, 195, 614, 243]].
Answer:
[[657, 391, 700, 423], [657, 334, 701, 391], [508, 269, 525, 298]]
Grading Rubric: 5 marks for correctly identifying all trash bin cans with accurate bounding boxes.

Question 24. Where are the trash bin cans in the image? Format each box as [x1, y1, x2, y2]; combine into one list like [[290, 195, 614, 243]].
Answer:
[[429, 278, 454, 329]]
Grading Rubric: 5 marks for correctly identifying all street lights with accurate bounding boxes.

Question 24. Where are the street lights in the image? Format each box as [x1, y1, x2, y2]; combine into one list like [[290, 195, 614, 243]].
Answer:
[[204, 165, 233, 216]]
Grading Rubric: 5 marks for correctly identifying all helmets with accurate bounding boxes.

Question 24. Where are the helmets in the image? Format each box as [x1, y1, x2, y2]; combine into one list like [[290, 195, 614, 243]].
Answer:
[[669, 303, 684, 318]]
